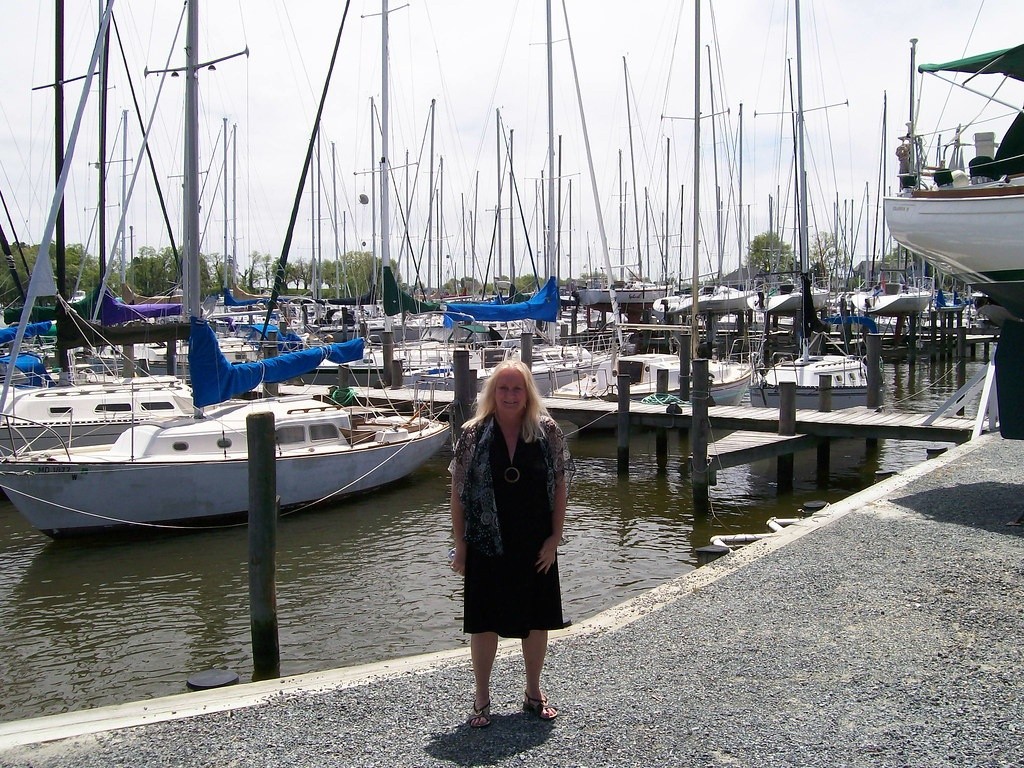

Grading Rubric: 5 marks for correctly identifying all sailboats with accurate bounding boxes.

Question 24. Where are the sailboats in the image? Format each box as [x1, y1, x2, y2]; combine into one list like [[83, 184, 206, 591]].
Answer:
[[0, 0, 1024, 537]]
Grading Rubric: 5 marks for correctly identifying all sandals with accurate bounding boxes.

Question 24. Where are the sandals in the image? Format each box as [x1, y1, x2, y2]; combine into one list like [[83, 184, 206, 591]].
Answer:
[[466, 700, 491, 726], [523, 691, 558, 719]]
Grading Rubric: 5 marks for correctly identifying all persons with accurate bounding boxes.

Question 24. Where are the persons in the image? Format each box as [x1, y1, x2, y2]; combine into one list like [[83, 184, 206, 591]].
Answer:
[[448, 358, 577, 729]]
